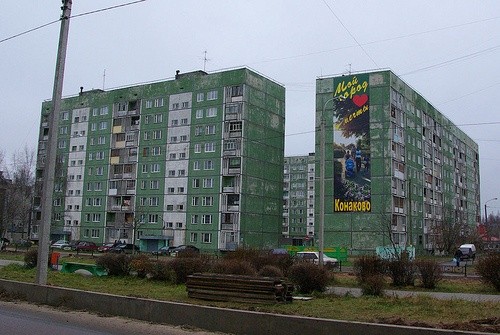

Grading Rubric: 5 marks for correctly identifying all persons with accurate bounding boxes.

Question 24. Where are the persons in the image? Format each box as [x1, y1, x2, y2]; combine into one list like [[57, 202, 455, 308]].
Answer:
[[364, 154, 370, 173], [113, 240, 117, 248], [351, 152, 355, 161], [134, 245, 140, 255], [468, 248, 473, 258], [351, 145, 356, 153], [345, 150, 351, 160], [454, 247, 462, 267], [117, 238, 126, 245], [355, 146, 362, 173]]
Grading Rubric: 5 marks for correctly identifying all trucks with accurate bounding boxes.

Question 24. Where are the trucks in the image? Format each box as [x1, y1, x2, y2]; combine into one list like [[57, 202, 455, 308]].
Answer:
[[459, 244, 476, 260]]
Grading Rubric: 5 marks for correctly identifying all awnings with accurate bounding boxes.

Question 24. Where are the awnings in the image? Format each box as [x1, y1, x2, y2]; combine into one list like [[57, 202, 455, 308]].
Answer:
[[292, 237, 307, 239]]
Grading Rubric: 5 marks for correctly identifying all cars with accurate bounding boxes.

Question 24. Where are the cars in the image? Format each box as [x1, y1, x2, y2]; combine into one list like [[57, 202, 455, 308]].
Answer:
[[296, 251, 338, 268], [97, 243, 118, 252], [51, 240, 97, 252], [152, 246, 176, 256], [168, 245, 201, 254], [112, 243, 137, 254]]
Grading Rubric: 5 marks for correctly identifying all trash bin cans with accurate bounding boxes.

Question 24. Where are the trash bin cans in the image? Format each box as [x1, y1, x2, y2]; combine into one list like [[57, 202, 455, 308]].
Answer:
[[50, 252, 60, 265]]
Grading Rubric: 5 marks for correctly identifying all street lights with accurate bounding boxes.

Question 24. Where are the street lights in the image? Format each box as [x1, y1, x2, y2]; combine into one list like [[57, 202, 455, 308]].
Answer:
[[317, 94, 346, 268], [484, 197, 497, 222]]
